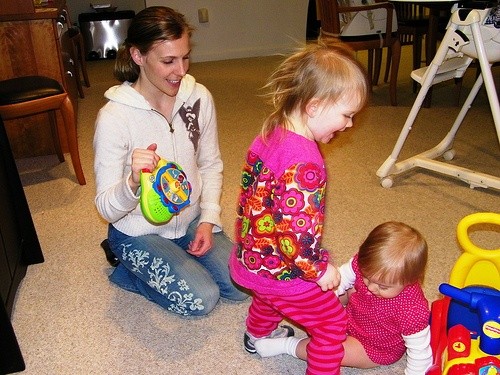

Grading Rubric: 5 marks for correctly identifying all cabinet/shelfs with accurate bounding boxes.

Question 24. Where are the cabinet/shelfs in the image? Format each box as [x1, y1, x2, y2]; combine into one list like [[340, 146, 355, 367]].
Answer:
[[0, 0, 77, 156], [0, 115, 46, 375]]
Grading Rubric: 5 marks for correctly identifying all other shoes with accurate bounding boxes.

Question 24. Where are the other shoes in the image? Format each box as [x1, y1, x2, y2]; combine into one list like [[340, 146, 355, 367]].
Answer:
[[243, 324, 294, 355]]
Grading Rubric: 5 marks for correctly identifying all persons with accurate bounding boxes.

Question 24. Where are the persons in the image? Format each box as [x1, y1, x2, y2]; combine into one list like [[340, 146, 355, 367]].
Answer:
[[254, 220, 432, 375], [228, 37, 368, 375], [93, 6, 253, 317]]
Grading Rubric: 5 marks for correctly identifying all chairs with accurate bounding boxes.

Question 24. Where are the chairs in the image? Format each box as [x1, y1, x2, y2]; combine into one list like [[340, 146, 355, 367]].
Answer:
[[376, 1, 500, 192], [305, 0, 500, 108], [0, 25, 90, 185]]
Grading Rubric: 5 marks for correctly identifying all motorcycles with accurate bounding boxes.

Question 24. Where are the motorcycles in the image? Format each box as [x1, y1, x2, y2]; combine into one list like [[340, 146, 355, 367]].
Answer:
[[425, 212, 500, 375]]
